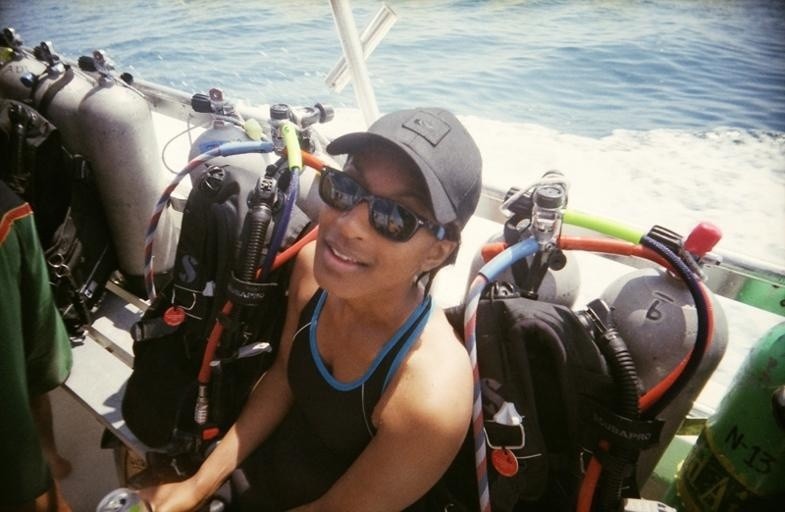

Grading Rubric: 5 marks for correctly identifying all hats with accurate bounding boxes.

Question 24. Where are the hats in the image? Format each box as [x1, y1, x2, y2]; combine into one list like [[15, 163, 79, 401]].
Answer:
[[326, 108, 482, 233]]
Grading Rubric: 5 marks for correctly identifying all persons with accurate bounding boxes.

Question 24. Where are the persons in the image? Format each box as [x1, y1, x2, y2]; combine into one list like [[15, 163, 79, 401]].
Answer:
[[0, 179, 77, 512], [138, 106, 479, 512]]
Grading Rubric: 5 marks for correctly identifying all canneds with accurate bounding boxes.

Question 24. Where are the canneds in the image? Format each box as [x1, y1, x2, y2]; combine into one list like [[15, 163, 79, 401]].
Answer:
[[96, 488, 151, 512]]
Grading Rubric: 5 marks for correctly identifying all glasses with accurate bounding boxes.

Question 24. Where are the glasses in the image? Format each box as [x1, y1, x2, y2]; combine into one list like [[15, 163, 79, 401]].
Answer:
[[319, 165, 457, 241]]
